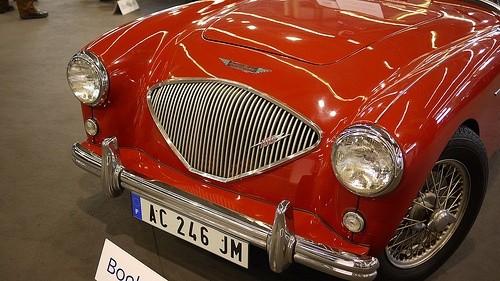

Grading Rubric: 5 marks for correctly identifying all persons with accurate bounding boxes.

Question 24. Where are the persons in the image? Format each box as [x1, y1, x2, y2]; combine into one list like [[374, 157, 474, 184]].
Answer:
[[0, 0, 48, 19]]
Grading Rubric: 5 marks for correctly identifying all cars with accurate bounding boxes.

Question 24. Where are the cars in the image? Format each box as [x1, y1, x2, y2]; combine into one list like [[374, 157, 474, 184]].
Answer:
[[65, 0, 500, 281]]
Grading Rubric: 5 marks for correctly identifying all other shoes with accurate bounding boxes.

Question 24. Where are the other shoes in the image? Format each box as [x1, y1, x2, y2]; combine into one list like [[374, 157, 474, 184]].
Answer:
[[20, 9, 48, 19], [0, 5, 15, 13]]
